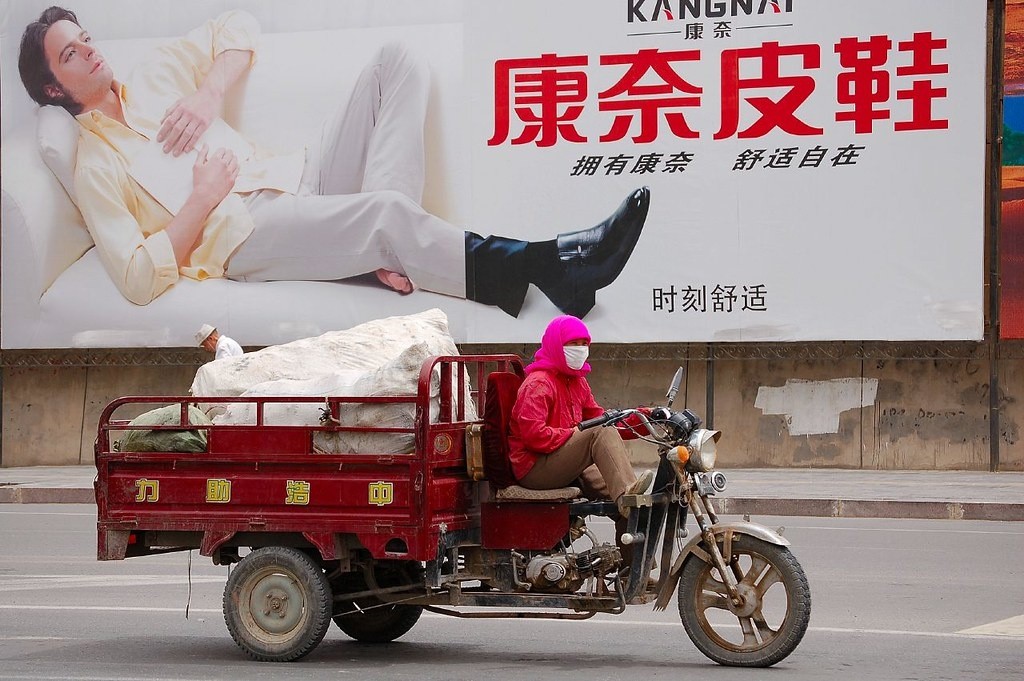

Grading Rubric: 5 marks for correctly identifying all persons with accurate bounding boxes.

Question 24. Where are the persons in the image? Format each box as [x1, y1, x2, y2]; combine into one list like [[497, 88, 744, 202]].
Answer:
[[195, 324, 243, 360], [17, 7, 650, 319], [507, 315, 663, 591]]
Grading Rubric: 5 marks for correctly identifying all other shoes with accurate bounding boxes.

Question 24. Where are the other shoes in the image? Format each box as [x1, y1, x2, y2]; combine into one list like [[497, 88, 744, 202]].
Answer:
[[613, 577, 658, 592], [617, 470, 653, 517]]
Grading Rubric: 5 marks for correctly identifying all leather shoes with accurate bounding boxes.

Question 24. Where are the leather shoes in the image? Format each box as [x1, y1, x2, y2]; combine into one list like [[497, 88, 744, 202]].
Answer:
[[546, 187, 651, 321]]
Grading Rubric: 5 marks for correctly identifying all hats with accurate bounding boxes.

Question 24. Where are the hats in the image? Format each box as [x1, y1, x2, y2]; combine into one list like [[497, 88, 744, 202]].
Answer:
[[194, 323, 218, 348]]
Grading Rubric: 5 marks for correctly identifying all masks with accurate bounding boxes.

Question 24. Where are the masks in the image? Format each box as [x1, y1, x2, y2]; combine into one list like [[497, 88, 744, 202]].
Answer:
[[562, 347, 591, 371]]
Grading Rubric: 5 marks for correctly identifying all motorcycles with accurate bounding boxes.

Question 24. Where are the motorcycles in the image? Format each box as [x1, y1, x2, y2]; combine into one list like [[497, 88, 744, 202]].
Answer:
[[91, 353, 813, 668]]
